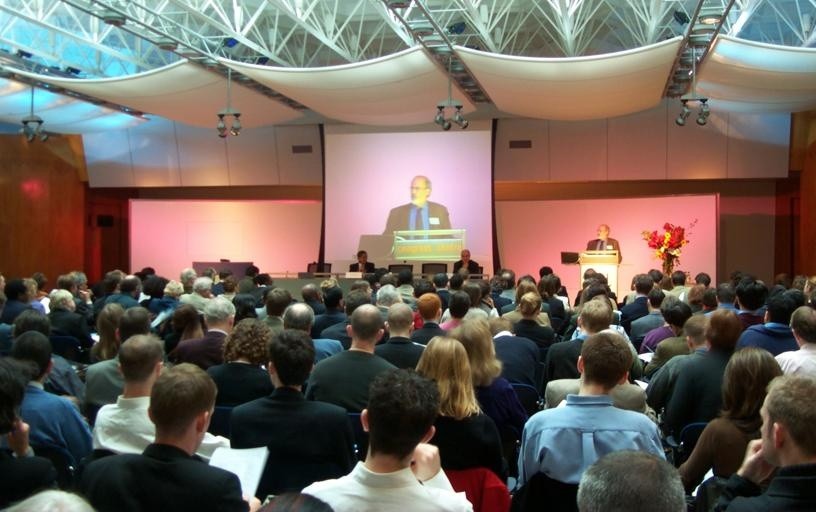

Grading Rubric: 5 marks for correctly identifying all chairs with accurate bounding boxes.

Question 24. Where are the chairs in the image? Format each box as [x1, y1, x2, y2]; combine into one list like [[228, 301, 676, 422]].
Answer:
[[387, 263, 413, 277], [50, 334, 88, 364], [348, 380, 715, 512], [477, 266, 483, 279], [307, 262, 333, 279], [420, 263, 449, 276], [205, 405, 236, 434]]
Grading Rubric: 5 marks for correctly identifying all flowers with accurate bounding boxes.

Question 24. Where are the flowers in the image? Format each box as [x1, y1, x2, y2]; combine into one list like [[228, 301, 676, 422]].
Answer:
[[640, 216, 704, 271]]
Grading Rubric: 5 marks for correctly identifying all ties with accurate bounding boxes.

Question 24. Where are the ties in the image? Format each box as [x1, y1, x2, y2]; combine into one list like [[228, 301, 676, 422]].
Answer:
[[415, 208, 423, 240], [361, 264, 363, 272], [599, 241, 605, 250]]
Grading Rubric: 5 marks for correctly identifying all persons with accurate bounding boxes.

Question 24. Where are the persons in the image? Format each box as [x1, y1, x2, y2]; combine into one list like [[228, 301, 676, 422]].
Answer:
[[349, 251, 375, 273], [2, 267, 816, 511], [382, 176, 452, 239], [586, 225, 622, 265], [453, 249, 479, 274]]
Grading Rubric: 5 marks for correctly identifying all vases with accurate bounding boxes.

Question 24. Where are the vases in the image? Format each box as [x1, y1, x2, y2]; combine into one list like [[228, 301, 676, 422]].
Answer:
[[662, 260, 674, 282]]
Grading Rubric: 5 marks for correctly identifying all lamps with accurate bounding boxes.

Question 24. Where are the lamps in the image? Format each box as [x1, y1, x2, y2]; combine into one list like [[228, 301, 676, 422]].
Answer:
[[432, 19, 469, 133], [18, 80, 52, 146], [216, 38, 243, 139], [669, 9, 719, 126]]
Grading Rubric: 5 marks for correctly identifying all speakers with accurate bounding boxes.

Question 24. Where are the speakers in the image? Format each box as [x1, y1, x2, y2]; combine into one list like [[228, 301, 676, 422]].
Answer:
[[96, 214, 114, 227]]
